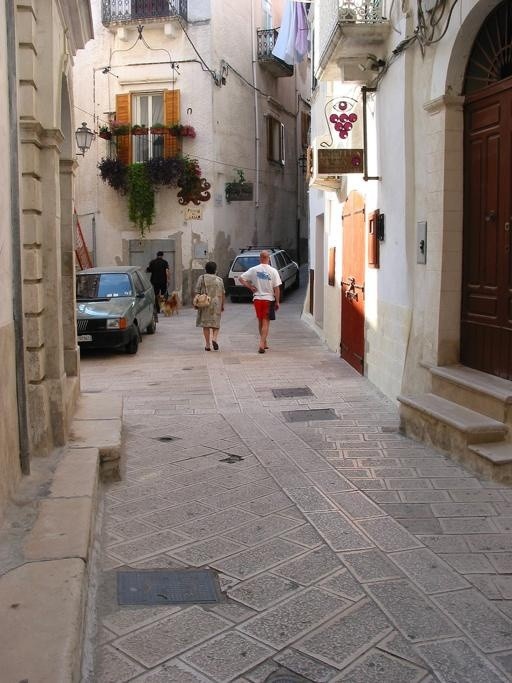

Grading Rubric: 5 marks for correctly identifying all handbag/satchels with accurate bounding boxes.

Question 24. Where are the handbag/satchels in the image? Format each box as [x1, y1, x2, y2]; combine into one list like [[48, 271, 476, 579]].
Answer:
[[193, 294, 211, 308]]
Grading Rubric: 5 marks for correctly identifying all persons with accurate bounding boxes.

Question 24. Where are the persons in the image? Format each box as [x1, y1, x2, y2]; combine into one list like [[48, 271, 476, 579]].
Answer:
[[144, 249, 171, 314], [236, 249, 284, 354], [191, 260, 226, 351]]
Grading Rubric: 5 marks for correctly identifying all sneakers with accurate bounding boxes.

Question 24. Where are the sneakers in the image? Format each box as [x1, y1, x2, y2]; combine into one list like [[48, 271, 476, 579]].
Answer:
[[205, 340, 218, 351]]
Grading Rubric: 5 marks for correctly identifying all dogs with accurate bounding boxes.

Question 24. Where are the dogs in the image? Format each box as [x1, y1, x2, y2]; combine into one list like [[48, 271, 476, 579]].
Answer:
[[160, 291, 179, 318]]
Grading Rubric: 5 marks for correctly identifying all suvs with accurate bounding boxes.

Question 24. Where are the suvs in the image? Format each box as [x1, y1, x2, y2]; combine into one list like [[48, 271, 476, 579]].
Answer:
[[227, 246, 300, 304]]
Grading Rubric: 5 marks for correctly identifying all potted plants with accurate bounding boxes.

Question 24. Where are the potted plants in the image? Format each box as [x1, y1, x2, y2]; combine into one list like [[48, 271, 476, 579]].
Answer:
[[98, 123, 198, 139]]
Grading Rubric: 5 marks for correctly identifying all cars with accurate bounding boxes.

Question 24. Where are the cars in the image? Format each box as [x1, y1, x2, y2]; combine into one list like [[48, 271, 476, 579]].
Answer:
[[76, 265, 158, 355]]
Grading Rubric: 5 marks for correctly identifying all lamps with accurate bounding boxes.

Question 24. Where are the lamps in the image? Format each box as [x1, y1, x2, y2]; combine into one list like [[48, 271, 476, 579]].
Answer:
[[74, 121, 96, 157]]
